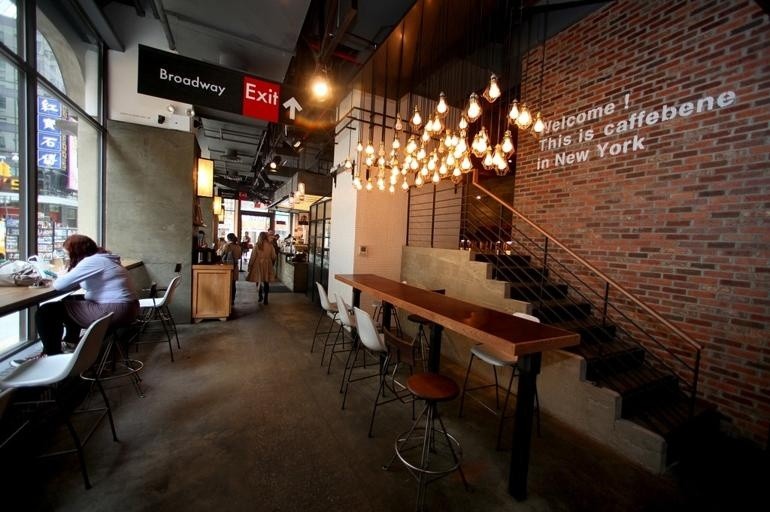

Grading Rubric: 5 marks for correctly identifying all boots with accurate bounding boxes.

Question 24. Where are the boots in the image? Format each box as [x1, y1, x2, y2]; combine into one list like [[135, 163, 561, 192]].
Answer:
[[264, 293, 268, 304], [258, 288, 263, 302]]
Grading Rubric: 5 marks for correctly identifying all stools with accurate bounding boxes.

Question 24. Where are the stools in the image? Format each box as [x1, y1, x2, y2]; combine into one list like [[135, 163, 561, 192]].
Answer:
[[381, 373, 474, 511]]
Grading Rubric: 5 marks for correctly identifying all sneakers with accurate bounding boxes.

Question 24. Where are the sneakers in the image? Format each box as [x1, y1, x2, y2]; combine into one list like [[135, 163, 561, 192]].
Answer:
[[61, 341, 76, 352], [10, 352, 47, 367]]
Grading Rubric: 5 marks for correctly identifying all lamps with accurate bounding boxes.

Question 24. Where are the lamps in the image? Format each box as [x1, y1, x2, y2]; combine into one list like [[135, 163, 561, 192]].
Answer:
[[269, 155, 281, 169], [197, 157, 214, 198], [345, 1, 546, 194]]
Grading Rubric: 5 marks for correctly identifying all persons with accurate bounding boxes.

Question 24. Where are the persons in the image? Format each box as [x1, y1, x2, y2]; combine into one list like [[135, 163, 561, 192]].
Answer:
[[217, 233, 243, 305], [193, 227, 280, 269], [11, 232, 140, 367], [245, 232, 276, 305]]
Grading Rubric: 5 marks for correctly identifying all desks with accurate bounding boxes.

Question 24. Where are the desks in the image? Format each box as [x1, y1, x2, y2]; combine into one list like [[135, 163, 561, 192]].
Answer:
[[335, 273, 581, 502], [0, 253, 145, 316]]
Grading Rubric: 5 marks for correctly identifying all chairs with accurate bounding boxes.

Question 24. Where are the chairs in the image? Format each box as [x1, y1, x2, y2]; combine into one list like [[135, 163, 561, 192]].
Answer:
[[0, 276, 182, 491], [311, 281, 541, 452]]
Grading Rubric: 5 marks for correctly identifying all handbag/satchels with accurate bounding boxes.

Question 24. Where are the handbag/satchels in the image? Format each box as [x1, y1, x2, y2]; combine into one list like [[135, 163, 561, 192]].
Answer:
[[0, 260, 42, 286]]
